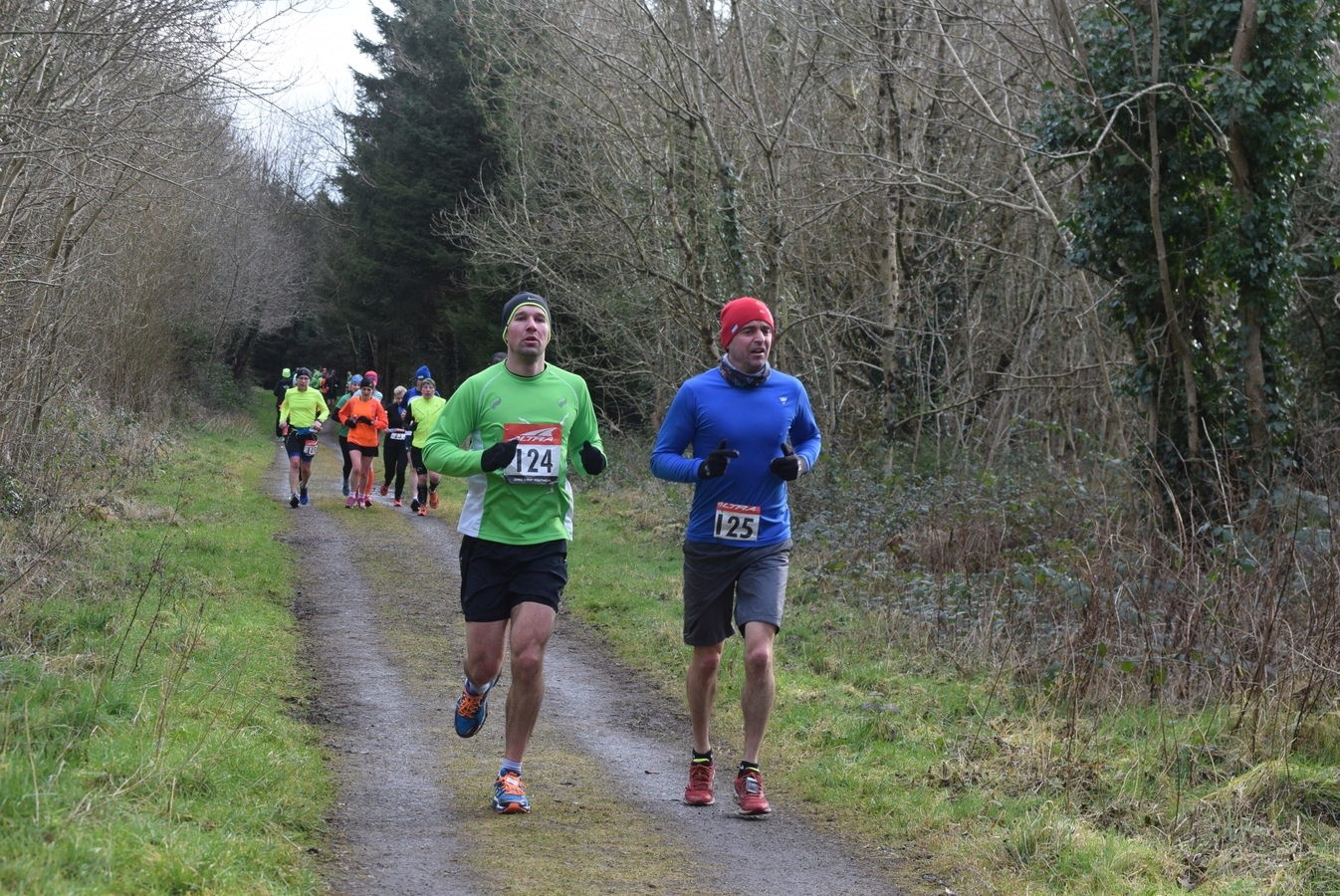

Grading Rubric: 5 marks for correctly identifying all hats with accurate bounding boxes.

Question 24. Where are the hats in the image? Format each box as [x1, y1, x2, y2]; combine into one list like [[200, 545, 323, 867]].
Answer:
[[502, 291, 551, 346], [282, 368, 291, 378], [351, 371, 377, 390], [415, 366, 431, 381], [296, 369, 311, 380], [719, 297, 775, 350]]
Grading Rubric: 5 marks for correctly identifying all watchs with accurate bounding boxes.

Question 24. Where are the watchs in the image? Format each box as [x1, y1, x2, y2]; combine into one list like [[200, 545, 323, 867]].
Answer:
[[315, 419, 323, 423]]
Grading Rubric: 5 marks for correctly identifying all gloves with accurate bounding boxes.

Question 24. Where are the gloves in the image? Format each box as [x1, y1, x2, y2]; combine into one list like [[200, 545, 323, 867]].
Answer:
[[345, 419, 357, 428], [770, 442, 808, 481], [698, 438, 740, 479], [356, 415, 373, 426], [578, 440, 607, 476], [480, 437, 519, 473]]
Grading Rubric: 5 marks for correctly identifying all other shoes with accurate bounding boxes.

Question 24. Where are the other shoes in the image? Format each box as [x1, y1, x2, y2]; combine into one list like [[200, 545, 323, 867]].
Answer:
[[411, 498, 420, 512], [417, 483, 427, 504], [343, 478, 373, 509]]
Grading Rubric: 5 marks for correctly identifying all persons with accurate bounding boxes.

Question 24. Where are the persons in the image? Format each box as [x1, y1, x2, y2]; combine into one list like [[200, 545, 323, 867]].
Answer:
[[399, 366, 440, 512], [274, 366, 383, 507], [380, 385, 409, 506], [339, 378, 389, 509], [422, 292, 609, 812], [466, 352, 508, 450], [404, 378, 447, 516], [649, 296, 822, 815]]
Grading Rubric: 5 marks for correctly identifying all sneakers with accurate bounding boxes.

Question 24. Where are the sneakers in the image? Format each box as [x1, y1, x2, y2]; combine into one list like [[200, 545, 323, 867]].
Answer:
[[453, 668, 501, 738], [394, 499, 401, 506], [289, 493, 299, 508], [732, 771, 771, 815], [416, 505, 428, 517], [298, 481, 308, 505], [381, 484, 388, 496], [684, 762, 715, 806], [428, 485, 439, 509], [492, 769, 531, 815]]
